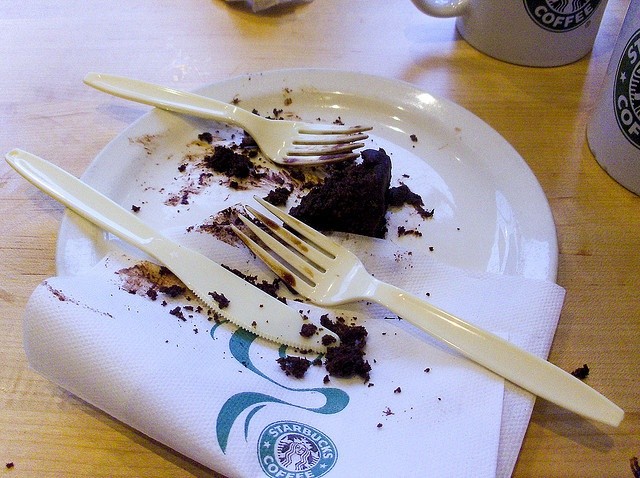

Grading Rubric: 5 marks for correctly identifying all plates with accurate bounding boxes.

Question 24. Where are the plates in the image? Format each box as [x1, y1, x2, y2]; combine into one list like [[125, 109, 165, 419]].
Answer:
[[56, 70, 559, 285]]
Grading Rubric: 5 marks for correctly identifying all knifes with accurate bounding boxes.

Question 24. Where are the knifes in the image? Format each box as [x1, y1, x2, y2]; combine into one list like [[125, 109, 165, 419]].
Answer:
[[5, 148, 341, 354]]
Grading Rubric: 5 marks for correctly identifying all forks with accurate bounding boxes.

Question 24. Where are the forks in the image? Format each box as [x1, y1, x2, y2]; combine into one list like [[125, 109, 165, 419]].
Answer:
[[229, 194, 625, 428], [84, 72, 372, 167]]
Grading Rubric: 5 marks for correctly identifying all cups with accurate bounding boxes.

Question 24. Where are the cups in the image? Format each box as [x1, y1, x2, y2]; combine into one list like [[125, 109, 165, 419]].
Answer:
[[586, 0, 640, 200], [413, 0, 609, 67]]
